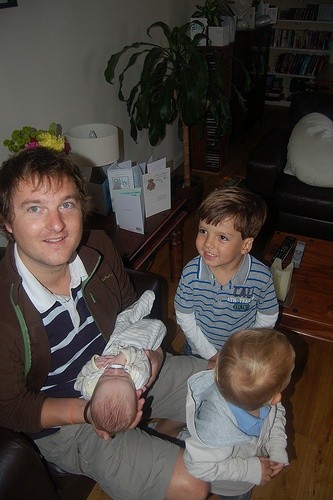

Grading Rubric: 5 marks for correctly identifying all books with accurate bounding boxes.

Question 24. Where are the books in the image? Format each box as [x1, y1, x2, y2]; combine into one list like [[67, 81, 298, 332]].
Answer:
[[274, 52, 330, 76], [205, 56, 220, 168], [288, 4, 320, 21], [265, 89, 285, 102], [271, 28, 333, 51]]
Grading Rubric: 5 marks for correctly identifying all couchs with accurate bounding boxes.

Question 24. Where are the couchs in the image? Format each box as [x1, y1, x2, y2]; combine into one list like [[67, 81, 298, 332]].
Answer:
[[0, 247, 169, 500], [245, 93, 333, 241]]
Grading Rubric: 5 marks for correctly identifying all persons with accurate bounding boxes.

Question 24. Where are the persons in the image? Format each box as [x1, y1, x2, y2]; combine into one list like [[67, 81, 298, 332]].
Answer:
[[183, 327, 296, 500], [173, 186, 279, 362], [0, 146, 216, 500], [74, 290, 167, 433]]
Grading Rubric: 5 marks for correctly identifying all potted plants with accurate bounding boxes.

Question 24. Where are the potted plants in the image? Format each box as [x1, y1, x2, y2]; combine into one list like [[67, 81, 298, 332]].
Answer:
[[104, 20, 251, 217], [191, 0, 230, 47]]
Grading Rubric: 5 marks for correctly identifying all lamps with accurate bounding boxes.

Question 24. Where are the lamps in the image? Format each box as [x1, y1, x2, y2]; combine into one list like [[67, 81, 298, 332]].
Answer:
[[67, 123, 120, 184]]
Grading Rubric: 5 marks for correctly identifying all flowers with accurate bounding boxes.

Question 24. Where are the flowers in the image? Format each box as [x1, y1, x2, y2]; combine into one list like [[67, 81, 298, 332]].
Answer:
[[4, 124, 72, 155]]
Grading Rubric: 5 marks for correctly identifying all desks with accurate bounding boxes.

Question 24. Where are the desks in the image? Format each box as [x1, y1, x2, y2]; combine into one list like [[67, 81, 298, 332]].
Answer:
[[84, 196, 188, 284], [258, 229, 333, 347]]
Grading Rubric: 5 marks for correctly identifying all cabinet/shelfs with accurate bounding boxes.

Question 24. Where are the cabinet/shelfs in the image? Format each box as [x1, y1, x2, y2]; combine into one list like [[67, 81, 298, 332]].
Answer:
[[192, 0, 333, 173]]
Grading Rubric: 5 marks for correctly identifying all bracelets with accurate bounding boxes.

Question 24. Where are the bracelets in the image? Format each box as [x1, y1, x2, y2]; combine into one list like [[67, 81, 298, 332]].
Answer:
[[83, 401, 92, 424]]
